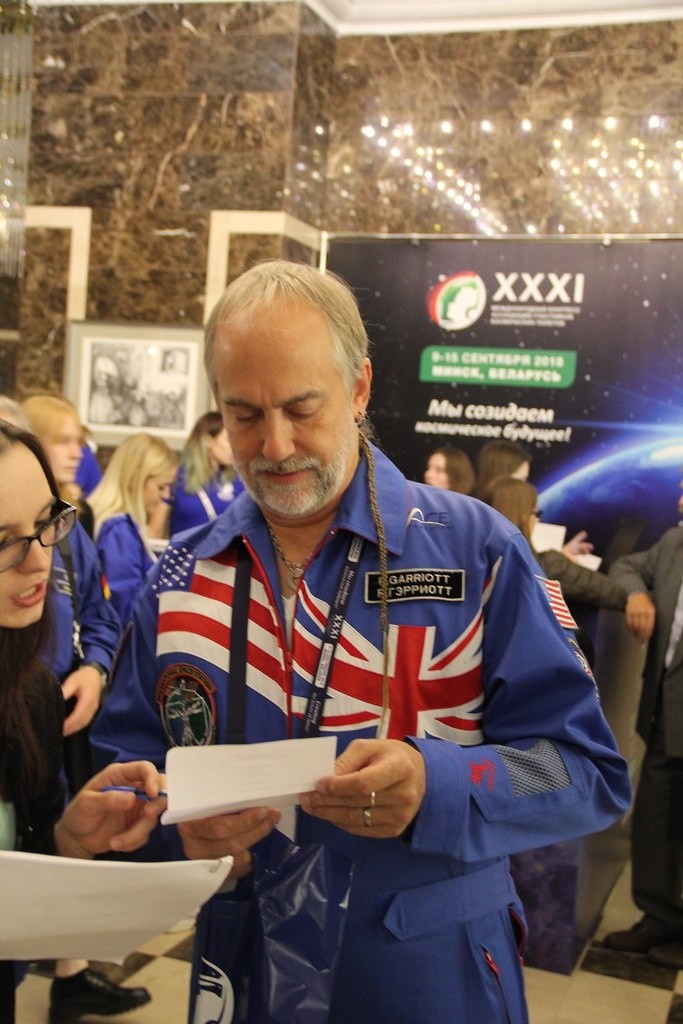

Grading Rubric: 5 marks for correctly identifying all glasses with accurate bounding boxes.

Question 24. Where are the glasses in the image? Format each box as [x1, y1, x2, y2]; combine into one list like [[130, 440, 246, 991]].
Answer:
[[0, 498, 77, 574]]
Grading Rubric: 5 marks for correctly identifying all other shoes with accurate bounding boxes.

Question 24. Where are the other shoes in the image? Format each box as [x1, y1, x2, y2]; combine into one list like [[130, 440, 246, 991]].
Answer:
[[648, 939, 683, 969], [605, 914, 674, 952]]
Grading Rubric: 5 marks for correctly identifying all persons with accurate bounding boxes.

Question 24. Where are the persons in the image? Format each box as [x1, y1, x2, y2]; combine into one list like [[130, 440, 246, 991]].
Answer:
[[89, 260, 632, 1023], [0, 420, 167, 1024], [0, 395, 244, 1024], [421, 433, 682, 968]]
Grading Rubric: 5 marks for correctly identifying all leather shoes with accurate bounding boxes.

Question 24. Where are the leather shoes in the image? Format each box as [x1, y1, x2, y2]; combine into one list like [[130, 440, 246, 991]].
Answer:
[[48, 967, 151, 1023]]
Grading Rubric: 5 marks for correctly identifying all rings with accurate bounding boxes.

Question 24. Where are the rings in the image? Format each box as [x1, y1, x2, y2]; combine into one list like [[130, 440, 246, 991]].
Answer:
[[371, 792, 375, 808], [364, 807, 371, 827]]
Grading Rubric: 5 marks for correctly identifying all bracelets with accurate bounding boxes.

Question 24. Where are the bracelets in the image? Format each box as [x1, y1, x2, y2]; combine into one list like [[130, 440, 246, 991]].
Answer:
[[91, 661, 107, 689]]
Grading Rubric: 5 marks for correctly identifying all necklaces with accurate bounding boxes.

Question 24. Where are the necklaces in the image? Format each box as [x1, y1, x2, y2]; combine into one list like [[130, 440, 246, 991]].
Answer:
[[268, 525, 330, 591]]
